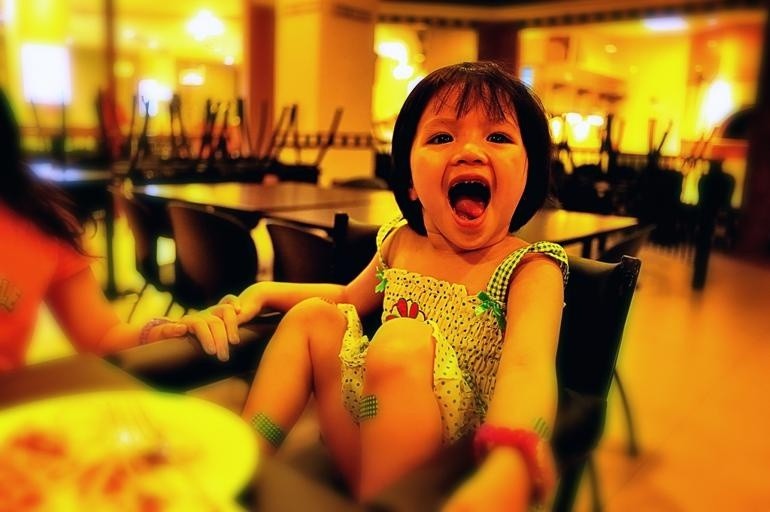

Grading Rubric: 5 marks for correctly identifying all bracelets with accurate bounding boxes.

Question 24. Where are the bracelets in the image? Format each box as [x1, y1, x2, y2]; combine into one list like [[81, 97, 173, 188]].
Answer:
[[467, 421, 563, 506], [139, 316, 176, 345]]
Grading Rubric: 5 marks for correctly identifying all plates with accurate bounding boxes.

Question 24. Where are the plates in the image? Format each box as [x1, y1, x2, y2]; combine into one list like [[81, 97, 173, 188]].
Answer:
[[1, 388, 258, 512]]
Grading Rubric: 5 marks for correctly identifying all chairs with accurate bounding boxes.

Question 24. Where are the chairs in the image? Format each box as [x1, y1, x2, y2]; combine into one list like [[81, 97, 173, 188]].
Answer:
[[107, 212, 657, 512]]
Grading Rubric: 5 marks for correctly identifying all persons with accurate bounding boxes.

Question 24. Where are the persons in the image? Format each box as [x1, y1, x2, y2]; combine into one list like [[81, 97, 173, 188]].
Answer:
[[223, 61, 573, 511], [0, 86, 244, 375]]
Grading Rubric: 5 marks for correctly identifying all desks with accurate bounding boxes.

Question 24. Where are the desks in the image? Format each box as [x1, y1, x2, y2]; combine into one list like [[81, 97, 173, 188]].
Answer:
[[23, 95, 712, 301]]
